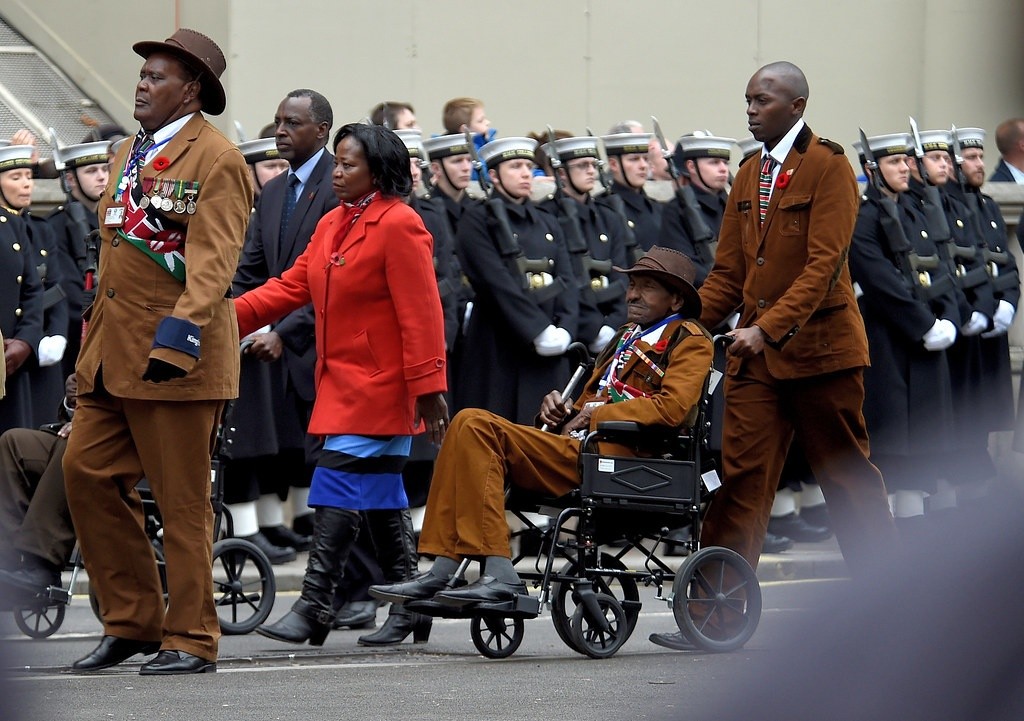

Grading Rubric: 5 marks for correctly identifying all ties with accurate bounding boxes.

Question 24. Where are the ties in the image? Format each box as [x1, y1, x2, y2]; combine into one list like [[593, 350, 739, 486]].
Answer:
[[135, 136, 155, 181], [759, 156, 778, 233], [279, 173, 301, 256], [616, 331, 641, 378]]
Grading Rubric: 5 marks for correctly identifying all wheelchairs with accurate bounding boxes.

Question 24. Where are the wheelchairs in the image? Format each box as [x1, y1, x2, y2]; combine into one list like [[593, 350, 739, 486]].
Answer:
[[8, 329, 283, 643], [445, 329, 762, 651]]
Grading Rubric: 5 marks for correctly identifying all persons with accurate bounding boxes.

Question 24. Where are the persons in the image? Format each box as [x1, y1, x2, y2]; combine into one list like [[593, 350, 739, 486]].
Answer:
[[648, 61, 912, 651], [236, 114, 453, 648], [0, 87, 1022, 567], [60, 27, 258, 677], [367, 244, 715, 621]]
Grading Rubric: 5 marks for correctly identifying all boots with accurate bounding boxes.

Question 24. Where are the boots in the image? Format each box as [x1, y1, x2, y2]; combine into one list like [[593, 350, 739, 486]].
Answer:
[[252, 505, 362, 646], [357, 506, 433, 645]]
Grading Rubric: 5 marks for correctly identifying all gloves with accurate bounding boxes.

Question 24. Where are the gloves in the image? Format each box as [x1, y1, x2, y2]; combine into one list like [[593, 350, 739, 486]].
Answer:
[[142, 358, 188, 383], [533, 324, 571, 356], [922, 299, 1015, 351], [588, 325, 616, 354], [3, 335, 77, 405]]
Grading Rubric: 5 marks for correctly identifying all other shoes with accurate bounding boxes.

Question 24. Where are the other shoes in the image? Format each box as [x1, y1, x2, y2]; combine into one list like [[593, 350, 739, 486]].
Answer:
[[508, 507, 708, 565]]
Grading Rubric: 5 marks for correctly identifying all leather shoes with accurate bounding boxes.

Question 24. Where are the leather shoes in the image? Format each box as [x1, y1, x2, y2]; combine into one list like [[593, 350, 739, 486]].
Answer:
[[0, 565, 61, 595], [224, 511, 317, 564], [758, 502, 833, 554], [72, 635, 162, 672], [139, 649, 217, 675], [433, 574, 529, 609], [332, 598, 389, 629], [368, 570, 468, 605], [648, 619, 728, 651]]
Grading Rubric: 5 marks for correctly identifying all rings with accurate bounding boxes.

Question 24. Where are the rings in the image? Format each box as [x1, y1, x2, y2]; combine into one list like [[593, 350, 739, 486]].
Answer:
[[438, 418, 445, 427]]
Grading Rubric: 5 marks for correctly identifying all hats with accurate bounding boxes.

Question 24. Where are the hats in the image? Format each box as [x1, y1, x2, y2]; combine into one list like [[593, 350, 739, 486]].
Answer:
[[610, 244, 702, 318], [851, 127, 988, 164], [235, 137, 281, 164], [132, 28, 226, 116], [392, 128, 765, 170], [0, 122, 131, 172]]
